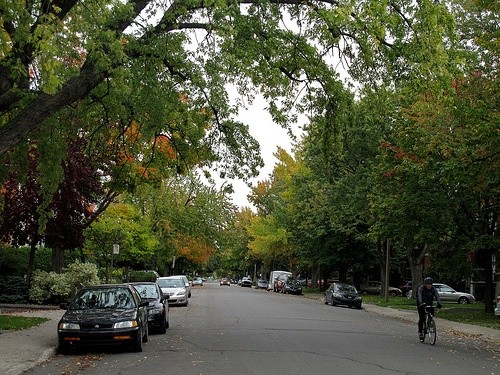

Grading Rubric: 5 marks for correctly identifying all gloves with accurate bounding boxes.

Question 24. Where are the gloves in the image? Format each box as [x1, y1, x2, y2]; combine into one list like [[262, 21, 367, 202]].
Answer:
[[421, 303, 426, 308], [437, 304, 442, 309]]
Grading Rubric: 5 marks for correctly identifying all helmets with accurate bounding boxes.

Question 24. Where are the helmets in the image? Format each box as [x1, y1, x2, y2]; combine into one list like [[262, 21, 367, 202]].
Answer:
[[425, 277, 434, 283]]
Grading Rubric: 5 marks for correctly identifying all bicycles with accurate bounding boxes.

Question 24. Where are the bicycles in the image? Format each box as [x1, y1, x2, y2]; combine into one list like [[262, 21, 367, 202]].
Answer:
[[419, 306, 440, 345]]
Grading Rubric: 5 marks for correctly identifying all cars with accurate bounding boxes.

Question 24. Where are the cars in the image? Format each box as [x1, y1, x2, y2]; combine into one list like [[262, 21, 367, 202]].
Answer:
[[399, 280, 413, 297], [237, 277, 253, 288], [57, 283, 149, 353], [255, 279, 268, 290], [123, 282, 170, 335], [493, 294, 500, 318], [156, 277, 189, 307], [324, 282, 362, 309], [219, 277, 230, 286], [432, 283, 476, 305], [201, 277, 207, 281], [229, 279, 237, 284], [192, 277, 203, 286], [169, 275, 192, 298]]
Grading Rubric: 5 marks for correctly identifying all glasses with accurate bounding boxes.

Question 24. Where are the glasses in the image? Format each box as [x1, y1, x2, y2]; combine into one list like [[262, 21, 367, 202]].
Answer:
[[427, 283, 432, 285]]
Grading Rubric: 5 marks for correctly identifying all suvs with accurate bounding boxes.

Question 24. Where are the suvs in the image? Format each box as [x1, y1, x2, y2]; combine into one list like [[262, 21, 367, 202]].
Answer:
[[360, 280, 403, 297], [274, 274, 302, 295]]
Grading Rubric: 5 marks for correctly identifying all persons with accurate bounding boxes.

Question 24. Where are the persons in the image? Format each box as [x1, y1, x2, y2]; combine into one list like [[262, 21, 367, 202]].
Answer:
[[416, 277, 443, 341], [400, 278, 408, 297]]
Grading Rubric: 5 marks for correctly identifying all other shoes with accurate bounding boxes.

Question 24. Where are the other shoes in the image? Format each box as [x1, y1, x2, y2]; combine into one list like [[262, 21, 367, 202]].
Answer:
[[427, 321, 433, 327]]
